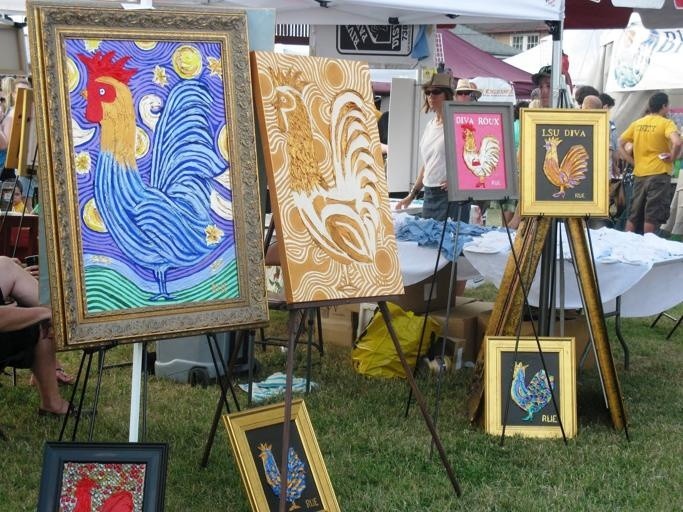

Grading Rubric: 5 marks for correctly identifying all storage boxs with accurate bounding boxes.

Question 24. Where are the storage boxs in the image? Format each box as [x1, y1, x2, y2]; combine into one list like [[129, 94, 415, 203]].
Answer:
[[312, 246, 594, 368]]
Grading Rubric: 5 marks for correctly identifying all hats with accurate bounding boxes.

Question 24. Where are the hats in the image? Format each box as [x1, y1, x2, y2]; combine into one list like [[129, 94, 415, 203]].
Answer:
[[454, 78, 481, 97], [422, 73, 454, 92], [532, 65, 552, 82]]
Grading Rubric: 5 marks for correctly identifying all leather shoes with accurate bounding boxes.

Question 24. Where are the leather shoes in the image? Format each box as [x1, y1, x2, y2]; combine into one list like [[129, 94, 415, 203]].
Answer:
[[38, 401, 97, 418]]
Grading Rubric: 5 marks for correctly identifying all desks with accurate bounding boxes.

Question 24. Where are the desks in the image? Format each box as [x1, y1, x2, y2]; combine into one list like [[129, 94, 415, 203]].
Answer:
[[474, 222, 683, 374]]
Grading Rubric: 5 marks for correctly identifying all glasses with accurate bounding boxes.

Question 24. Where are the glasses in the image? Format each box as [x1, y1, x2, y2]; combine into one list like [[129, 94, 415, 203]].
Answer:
[[424, 90, 444, 95], [456, 90, 472, 95]]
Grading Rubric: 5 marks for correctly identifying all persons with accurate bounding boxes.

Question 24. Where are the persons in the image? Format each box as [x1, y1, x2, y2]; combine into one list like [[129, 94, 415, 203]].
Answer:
[[455, 68, 682, 233], [395, 73, 469, 226], [0, 63, 78, 418]]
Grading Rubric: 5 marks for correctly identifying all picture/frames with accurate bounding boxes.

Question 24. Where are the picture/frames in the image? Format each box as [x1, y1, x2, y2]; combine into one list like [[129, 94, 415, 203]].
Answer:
[[223, 399, 341, 511], [520, 106, 610, 217], [482, 333, 576, 441], [441, 100, 520, 204], [26, 0, 269, 353], [38, 439, 168, 512]]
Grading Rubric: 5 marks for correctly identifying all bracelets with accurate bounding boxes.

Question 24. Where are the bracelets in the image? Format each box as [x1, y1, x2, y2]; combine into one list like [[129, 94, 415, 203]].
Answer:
[[410, 187, 419, 195]]
